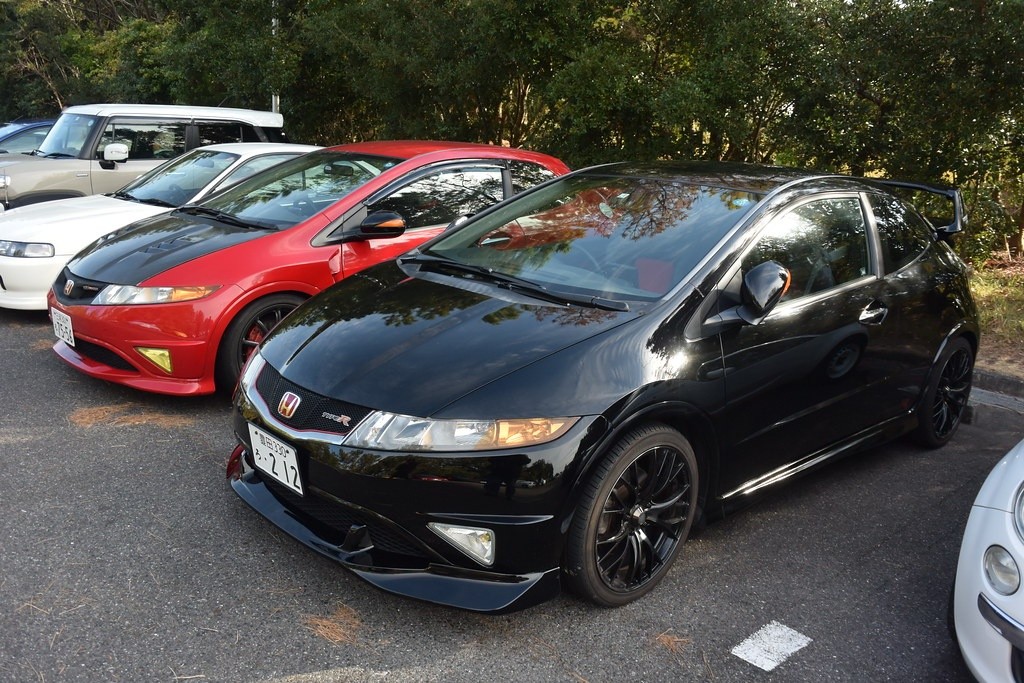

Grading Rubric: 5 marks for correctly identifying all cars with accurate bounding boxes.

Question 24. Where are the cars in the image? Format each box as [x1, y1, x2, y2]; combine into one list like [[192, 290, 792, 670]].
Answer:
[[0, 117, 58, 156], [953, 440, 1024, 683]]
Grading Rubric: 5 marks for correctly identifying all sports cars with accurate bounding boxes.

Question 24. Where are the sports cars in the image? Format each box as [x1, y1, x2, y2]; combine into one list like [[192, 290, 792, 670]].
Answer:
[[45, 140, 594, 405], [220, 157, 983, 614], [0, 140, 383, 312]]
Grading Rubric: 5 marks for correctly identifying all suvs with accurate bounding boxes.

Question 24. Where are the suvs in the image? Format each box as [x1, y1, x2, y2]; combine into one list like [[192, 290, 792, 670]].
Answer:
[[0, 102, 288, 216]]
